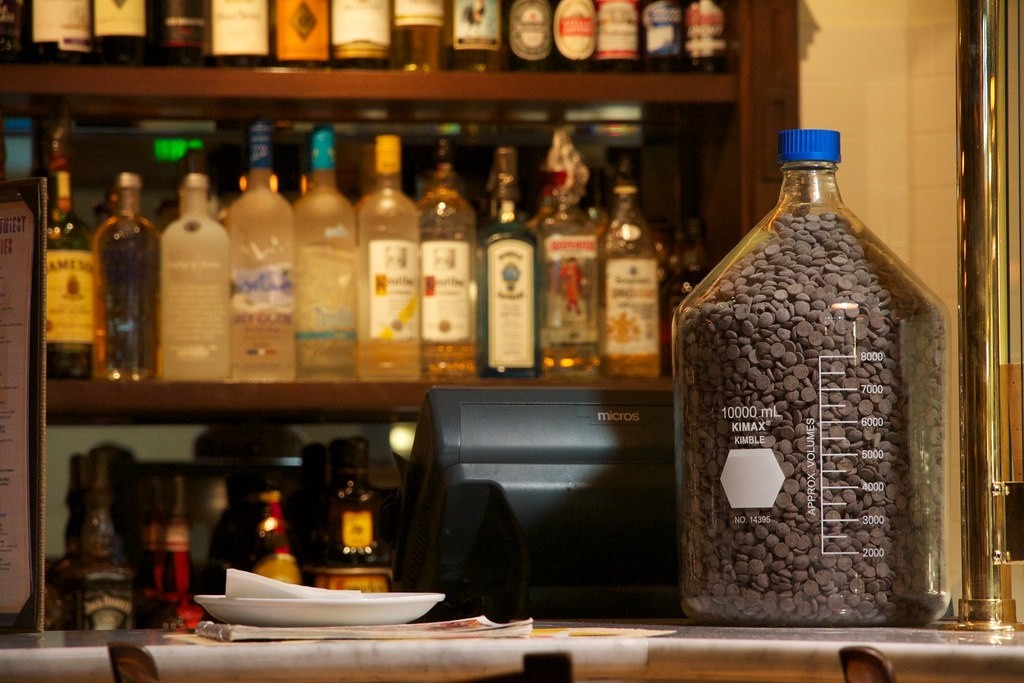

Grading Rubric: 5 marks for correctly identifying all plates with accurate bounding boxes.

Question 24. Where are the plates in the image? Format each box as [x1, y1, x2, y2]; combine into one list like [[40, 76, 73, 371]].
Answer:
[[193, 593, 445, 626]]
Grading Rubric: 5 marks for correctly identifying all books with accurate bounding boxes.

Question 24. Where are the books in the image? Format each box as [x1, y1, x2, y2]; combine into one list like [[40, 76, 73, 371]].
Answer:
[[197, 615, 535, 642]]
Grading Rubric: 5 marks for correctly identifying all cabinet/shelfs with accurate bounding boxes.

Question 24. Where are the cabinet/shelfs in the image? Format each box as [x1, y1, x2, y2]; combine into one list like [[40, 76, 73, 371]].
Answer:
[[0, 0, 805, 633]]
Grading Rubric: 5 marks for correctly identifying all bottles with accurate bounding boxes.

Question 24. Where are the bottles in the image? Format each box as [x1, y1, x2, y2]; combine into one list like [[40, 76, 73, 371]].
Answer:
[[1, 1, 946, 627]]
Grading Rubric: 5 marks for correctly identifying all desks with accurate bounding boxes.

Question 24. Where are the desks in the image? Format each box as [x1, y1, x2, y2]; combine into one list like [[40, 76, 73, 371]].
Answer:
[[0, 616, 1024, 683]]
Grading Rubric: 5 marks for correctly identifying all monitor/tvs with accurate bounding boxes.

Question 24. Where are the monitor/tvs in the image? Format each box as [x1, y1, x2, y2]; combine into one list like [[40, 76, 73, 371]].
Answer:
[[392, 389, 685, 618]]
